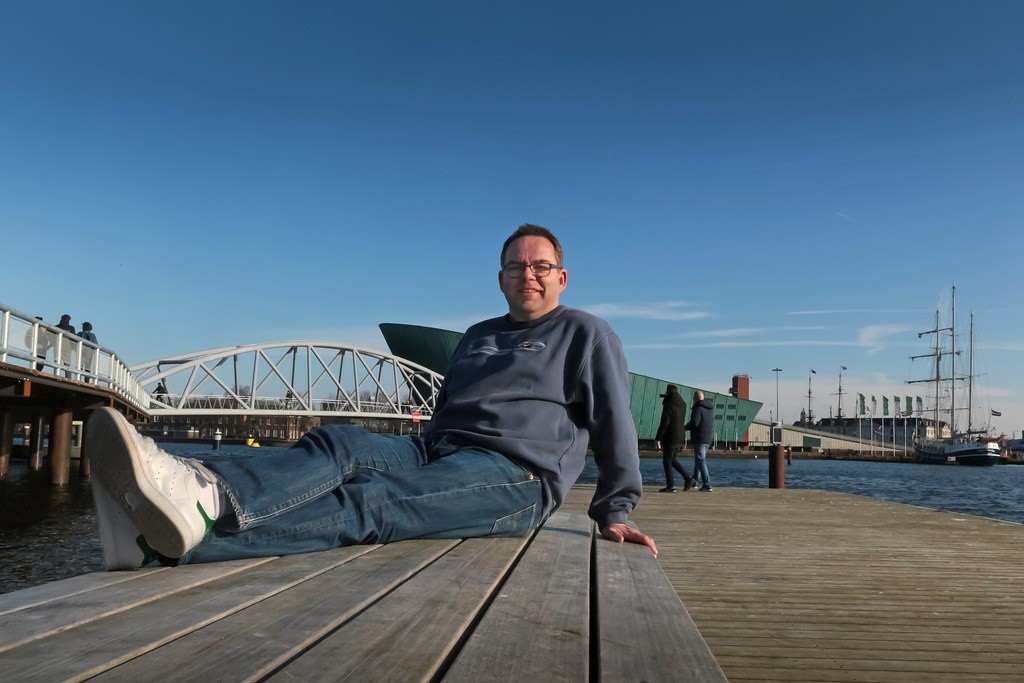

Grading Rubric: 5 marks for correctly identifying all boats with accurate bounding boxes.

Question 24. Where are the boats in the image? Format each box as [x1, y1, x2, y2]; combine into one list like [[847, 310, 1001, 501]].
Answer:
[[245, 428, 260, 448]]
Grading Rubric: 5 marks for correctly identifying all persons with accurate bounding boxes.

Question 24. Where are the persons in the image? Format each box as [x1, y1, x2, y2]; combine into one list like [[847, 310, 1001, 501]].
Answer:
[[84, 223, 659, 571], [51, 315, 75, 379], [654, 385, 695, 493], [395, 400, 413, 414], [286, 391, 291, 400], [152, 382, 164, 402], [74, 322, 98, 384], [25, 317, 52, 372], [684, 390, 715, 492]]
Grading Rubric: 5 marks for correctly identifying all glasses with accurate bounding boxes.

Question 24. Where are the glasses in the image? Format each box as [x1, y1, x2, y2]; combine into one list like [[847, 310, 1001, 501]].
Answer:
[[502, 263, 562, 279]]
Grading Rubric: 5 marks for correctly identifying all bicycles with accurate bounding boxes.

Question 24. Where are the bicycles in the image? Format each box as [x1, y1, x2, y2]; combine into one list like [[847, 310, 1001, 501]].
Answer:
[[279, 399, 293, 410], [150, 392, 167, 409]]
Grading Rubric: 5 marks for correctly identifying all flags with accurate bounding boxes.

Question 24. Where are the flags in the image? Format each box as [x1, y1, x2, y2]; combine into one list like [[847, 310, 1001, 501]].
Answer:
[[992, 410, 1001, 416], [812, 370, 816, 373], [842, 366, 847, 370]]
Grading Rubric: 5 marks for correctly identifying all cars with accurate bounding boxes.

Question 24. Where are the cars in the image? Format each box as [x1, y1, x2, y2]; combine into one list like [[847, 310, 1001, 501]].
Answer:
[[238, 432, 247, 438], [844, 449, 863, 457]]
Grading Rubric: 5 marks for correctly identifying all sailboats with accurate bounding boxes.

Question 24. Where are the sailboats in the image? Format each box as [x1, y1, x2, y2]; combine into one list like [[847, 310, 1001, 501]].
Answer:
[[903, 282, 1001, 465]]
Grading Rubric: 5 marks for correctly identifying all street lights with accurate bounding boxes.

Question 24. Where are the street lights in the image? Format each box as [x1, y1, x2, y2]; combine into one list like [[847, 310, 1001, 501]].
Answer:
[[400, 422, 407, 436], [772, 367, 783, 422]]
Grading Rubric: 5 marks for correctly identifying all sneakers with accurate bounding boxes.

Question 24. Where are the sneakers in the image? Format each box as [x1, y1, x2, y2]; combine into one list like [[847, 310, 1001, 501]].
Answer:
[[87, 406, 234, 558], [90, 473, 146, 570]]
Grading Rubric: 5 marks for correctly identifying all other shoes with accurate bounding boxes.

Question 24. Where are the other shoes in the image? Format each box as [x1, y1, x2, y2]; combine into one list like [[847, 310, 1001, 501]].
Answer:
[[659, 486, 676, 493], [684, 478, 695, 491], [691, 486, 697, 489], [699, 486, 712, 492]]
